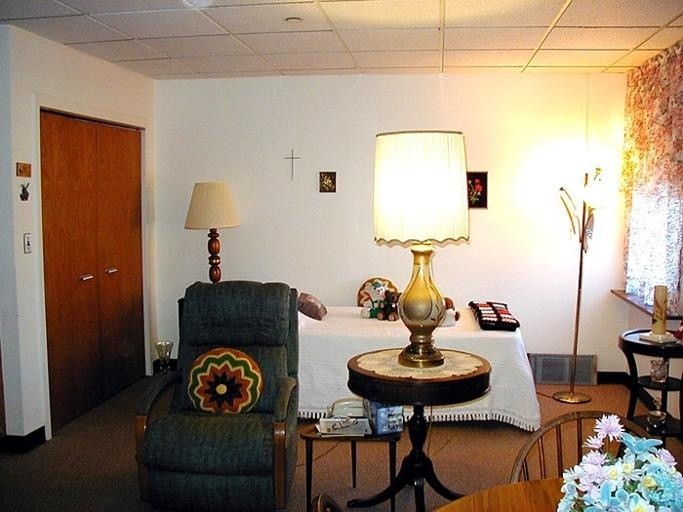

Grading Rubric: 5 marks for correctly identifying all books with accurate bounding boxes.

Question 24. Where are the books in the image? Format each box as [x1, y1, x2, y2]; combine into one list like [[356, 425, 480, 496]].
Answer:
[[314, 417, 373, 439]]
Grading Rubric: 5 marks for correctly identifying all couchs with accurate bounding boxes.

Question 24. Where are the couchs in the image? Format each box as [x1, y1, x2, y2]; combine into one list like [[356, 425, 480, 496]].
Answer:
[[133, 280, 297, 509]]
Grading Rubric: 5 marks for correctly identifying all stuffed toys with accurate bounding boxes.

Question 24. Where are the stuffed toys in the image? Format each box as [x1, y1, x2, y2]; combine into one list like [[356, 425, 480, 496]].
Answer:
[[378, 290, 402, 321], [442, 297, 461, 327]]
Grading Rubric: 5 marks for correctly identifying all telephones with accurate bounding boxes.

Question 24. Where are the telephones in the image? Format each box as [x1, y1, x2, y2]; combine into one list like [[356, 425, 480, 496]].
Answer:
[[327, 398, 364, 418]]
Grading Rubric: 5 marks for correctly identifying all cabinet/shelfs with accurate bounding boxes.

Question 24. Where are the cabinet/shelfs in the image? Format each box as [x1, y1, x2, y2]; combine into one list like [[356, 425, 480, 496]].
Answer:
[[616, 328, 683, 459]]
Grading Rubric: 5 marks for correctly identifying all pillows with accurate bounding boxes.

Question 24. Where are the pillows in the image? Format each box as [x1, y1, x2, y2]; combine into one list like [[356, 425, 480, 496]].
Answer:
[[298, 293, 327, 321], [185, 348, 264, 417]]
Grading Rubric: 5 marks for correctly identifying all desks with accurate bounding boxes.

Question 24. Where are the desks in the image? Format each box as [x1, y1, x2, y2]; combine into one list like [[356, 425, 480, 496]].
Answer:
[[301, 415, 399, 512], [432, 473, 683, 512], [298, 306, 537, 430], [346, 343, 492, 512]]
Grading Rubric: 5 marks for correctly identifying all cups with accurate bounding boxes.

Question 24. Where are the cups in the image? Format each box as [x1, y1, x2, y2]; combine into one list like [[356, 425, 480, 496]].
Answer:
[[649, 359, 669, 382]]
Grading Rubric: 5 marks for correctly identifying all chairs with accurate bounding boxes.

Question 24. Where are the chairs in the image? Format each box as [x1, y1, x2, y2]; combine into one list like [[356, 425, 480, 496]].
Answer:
[[509, 411, 649, 484]]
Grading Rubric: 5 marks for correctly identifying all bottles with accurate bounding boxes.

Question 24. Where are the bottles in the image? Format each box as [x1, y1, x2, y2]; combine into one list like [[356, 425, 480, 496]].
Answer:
[[646, 410, 667, 432]]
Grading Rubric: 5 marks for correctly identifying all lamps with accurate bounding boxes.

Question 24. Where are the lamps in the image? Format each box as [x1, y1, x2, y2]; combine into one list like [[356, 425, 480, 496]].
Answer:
[[551, 156, 609, 406], [373, 129, 471, 364], [185, 178, 241, 284]]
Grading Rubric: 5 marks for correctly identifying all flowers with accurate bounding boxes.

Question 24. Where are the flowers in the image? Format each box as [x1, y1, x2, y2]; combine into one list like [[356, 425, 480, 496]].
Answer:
[[552, 414, 683, 512]]
